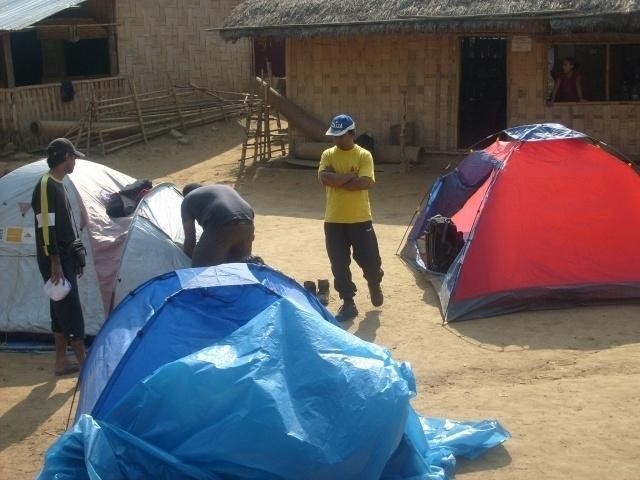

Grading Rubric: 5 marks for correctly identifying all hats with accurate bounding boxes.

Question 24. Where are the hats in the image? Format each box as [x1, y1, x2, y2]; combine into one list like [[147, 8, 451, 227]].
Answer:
[[48, 138, 85, 157], [325, 115, 355, 137]]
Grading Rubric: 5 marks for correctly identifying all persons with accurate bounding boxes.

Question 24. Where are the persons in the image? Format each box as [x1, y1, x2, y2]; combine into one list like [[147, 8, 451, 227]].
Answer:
[[180, 181, 257, 270], [31, 139, 88, 378], [547, 55, 588, 103], [318, 111, 385, 322]]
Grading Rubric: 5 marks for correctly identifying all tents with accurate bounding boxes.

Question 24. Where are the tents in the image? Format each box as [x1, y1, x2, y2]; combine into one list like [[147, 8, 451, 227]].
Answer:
[[394, 122, 639, 327], [64, 262, 434, 480], [0, 159, 204, 356]]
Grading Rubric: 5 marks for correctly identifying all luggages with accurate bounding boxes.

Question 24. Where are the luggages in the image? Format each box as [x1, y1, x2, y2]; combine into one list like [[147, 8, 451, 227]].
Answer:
[[425, 215, 457, 271]]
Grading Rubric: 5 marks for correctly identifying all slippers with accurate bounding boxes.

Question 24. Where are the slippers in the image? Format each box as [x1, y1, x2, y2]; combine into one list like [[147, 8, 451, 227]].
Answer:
[[55, 363, 80, 375]]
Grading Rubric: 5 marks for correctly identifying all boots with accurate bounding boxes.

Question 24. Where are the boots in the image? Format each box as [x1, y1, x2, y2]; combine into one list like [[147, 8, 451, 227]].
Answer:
[[335, 296, 358, 322], [316, 279, 329, 304], [304, 281, 316, 295], [368, 281, 382, 306]]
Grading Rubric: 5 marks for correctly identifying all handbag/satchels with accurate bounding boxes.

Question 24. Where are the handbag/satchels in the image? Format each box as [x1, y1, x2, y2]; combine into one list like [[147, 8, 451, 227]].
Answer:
[[58, 240, 86, 275]]
